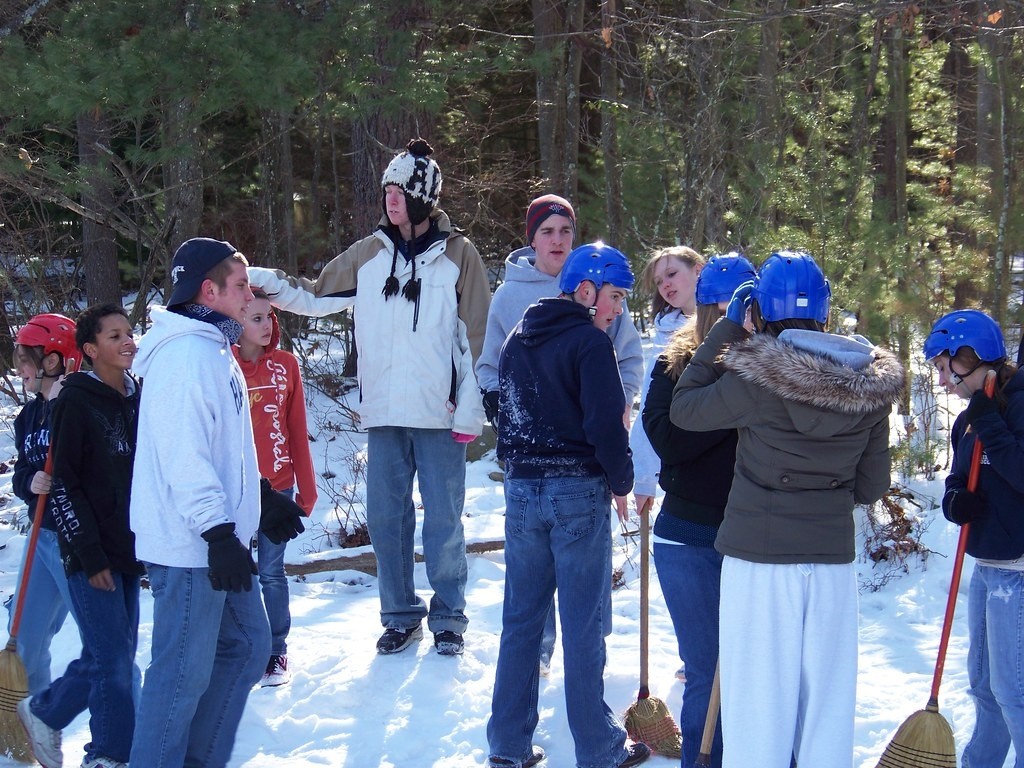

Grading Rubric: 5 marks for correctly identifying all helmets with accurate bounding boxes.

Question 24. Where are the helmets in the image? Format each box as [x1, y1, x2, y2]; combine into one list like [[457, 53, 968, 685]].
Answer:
[[558, 243, 634, 295], [694, 252, 756, 305], [922, 309, 1007, 364], [16, 313, 83, 375], [750, 251, 830, 323]]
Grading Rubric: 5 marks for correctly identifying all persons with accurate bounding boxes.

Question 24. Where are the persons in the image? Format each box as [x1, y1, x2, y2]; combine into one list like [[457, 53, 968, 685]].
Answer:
[[230, 286, 318, 688], [19, 303, 150, 768], [245, 138, 491, 655], [923, 310, 1024, 768], [670, 250, 905, 768], [130, 238, 306, 768], [628, 246, 706, 682], [641, 255, 757, 768], [475, 194, 644, 674], [486, 243, 651, 768], [7, 313, 83, 696]]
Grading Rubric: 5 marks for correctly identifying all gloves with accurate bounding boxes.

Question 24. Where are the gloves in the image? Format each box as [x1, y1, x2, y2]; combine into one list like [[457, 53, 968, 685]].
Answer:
[[202, 522, 259, 593], [728, 279, 756, 326], [967, 388, 994, 425], [952, 489, 987, 525], [259, 478, 307, 545], [452, 432, 476, 444]]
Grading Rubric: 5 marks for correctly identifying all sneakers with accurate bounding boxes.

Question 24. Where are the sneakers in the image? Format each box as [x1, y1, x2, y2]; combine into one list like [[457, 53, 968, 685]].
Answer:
[[435, 626, 464, 657], [81, 756, 127, 768], [376, 621, 424, 654], [16, 694, 64, 768], [261, 652, 288, 685]]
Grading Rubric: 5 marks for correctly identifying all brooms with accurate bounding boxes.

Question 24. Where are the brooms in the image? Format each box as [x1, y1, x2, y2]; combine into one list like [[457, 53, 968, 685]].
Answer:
[[622, 495, 683, 760], [873, 367, 997, 768], [0, 356, 76, 766], [693, 654, 720, 768]]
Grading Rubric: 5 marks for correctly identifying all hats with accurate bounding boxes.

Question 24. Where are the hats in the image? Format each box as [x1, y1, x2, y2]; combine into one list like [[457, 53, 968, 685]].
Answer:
[[380, 137, 442, 304], [526, 192, 575, 245], [165, 238, 237, 308]]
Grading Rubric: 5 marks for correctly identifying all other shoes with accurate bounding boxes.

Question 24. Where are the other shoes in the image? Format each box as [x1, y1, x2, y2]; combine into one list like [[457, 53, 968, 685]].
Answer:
[[613, 741, 652, 768], [491, 743, 544, 768]]
